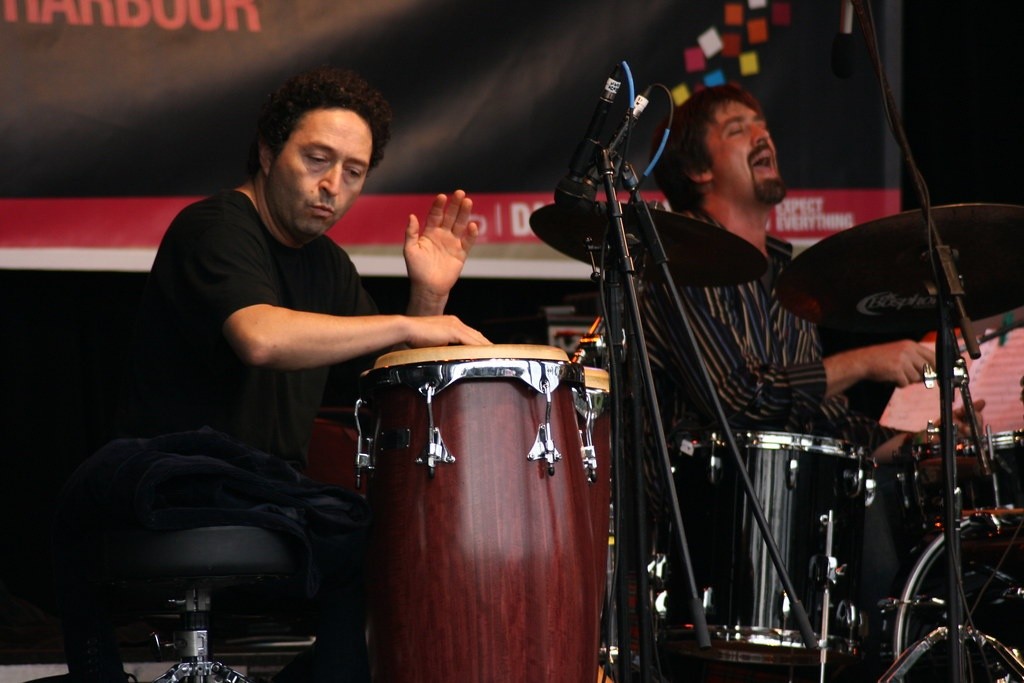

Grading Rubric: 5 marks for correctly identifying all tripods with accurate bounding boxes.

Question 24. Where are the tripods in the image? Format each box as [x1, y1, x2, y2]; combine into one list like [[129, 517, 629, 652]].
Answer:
[[852, 0, 1024, 683]]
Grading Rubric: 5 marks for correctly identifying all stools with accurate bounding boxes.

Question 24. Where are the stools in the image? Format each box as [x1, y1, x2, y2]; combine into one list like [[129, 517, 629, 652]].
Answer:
[[144, 524, 292, 683]]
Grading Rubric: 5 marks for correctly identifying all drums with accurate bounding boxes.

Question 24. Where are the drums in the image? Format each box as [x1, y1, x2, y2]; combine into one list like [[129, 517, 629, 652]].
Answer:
[[572, 363, 609, 610], [361, 342, 601, 683], [902, 427, 1024, 508], [885, 507, 1023, 683], [660, 428, 882, 666]]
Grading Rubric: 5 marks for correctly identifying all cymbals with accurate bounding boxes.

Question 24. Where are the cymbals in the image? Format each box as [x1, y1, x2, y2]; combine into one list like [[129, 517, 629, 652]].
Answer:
[[527, 199, 770, 291], [775, 202, 1023, 344]]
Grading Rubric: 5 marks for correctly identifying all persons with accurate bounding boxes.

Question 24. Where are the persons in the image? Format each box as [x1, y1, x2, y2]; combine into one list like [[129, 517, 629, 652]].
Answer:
[[632, 86, 936, 524], [55, 65, 494, 683]]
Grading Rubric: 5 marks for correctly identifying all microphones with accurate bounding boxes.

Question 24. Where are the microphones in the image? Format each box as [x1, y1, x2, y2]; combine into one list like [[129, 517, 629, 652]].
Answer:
[[839, 0, 854, 36], [553, 61, 654, 215]]
